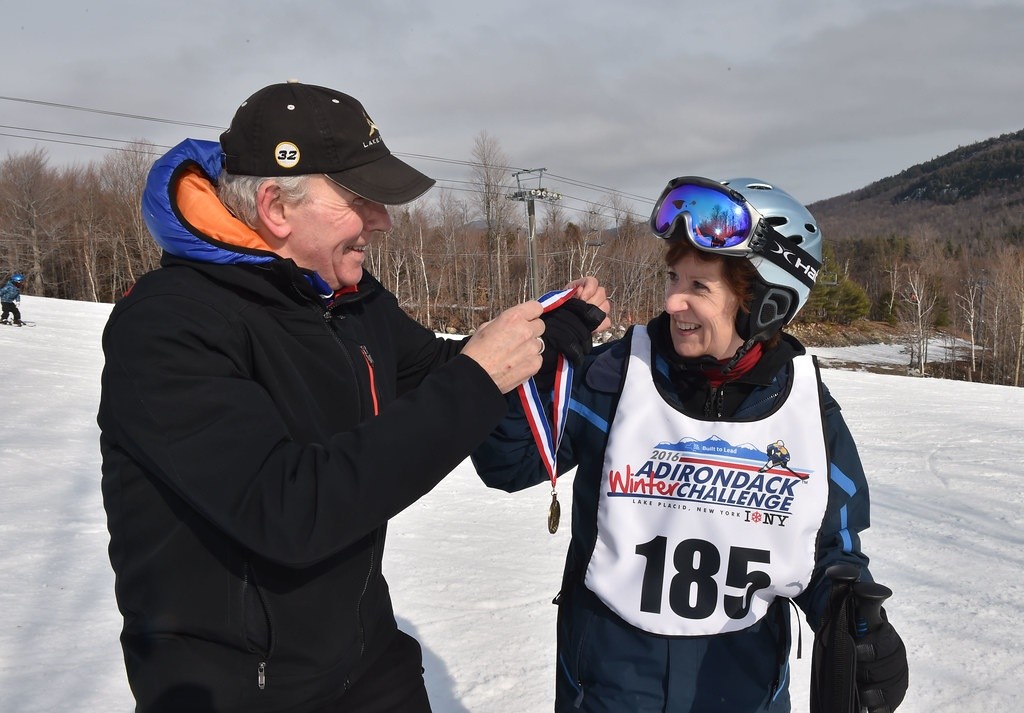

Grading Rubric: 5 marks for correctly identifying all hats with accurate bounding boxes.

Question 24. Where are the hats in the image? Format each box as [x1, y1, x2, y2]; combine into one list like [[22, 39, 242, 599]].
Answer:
[[218, 80, 437, 206]]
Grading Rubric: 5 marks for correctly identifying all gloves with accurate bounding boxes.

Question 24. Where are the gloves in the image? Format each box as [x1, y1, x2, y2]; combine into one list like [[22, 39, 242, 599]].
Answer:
[[818, 588, 909, 713], [540, 296, 606, 369]]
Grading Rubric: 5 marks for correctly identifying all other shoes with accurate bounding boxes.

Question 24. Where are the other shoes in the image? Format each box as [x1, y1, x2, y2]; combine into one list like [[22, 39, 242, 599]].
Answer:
[[0, 318, 12, 325], [14, 320, 26, 325]]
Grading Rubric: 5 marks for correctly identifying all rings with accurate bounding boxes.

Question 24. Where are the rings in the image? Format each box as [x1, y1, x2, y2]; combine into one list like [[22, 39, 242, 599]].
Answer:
[[536, 338, 545, 355]]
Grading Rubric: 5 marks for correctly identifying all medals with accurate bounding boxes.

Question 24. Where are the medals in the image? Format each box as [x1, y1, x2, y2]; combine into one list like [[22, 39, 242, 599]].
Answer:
[[548, 494, 562, 534]]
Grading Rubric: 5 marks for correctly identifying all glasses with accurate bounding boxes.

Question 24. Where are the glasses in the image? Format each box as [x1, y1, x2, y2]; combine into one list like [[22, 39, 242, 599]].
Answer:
[[650, 177, 822, 289]]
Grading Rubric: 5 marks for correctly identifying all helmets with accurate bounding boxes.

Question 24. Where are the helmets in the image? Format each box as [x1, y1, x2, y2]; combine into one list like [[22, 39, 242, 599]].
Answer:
[[11, 274, 24, 287], [647, 177, 827, 343]]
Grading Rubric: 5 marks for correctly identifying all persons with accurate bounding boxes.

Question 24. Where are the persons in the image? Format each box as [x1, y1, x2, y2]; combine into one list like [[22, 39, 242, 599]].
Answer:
[[471, 174, 908, 713], [0, 273, 25, 325], [97, 80, 611, 712]]
